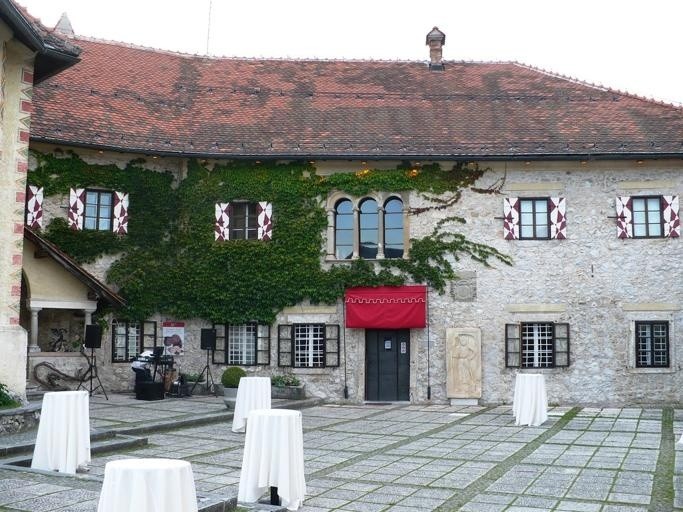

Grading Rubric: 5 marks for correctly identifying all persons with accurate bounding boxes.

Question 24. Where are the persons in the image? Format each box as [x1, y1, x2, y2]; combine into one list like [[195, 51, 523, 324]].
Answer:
[[131, 351, 153, 382]]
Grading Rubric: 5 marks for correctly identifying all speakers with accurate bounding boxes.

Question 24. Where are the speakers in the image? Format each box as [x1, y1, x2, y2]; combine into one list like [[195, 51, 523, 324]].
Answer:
[[85, 325, 102, 348], [201, 329, 216, 350]]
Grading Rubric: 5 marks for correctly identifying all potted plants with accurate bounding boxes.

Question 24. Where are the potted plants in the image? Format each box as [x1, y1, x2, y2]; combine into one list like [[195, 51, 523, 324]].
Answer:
[[219, 367, 247, 410], [271, 373, 307, 400]]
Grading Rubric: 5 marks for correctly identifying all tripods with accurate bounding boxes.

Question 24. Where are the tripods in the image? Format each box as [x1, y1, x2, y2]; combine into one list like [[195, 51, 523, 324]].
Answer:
[[190, 354, 218, 398], [76, 353, 109, 401]]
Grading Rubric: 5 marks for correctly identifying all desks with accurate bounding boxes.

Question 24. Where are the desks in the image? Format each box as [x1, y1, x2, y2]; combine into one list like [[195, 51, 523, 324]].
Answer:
[[513, 373, 548, 428], [32, 390, 93, 475], [105, 458, 197, 512], [233, 377, 302, 510]]
